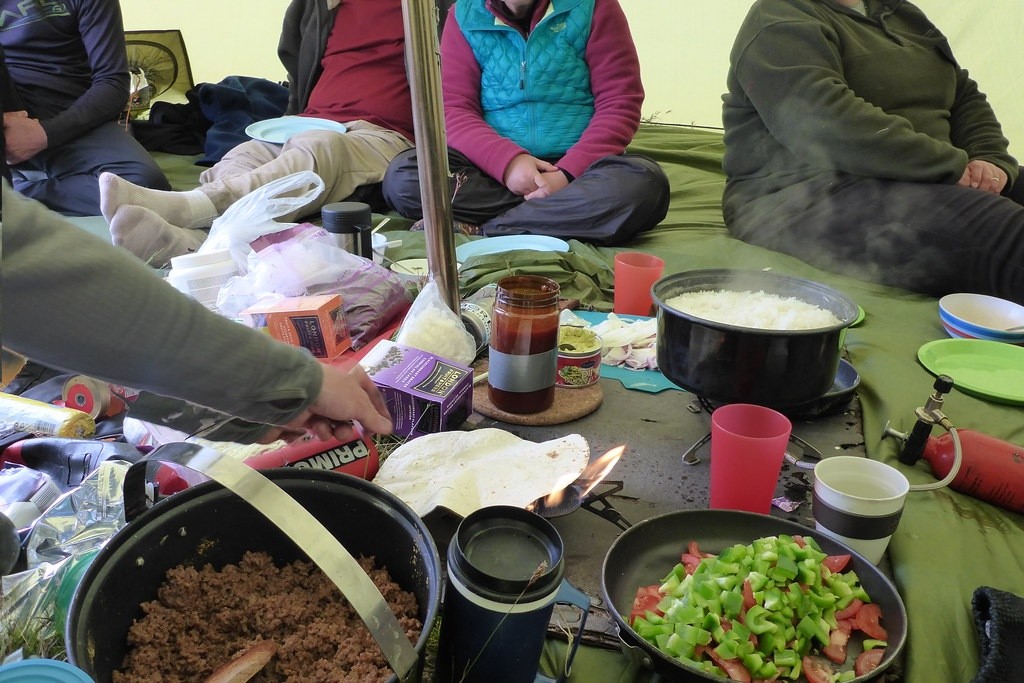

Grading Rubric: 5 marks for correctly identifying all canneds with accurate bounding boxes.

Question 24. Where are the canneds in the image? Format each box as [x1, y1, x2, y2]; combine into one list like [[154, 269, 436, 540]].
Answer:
[[486, 275, 561, 416], [556, 325, 602, 388], [62, 374, 142, 419]]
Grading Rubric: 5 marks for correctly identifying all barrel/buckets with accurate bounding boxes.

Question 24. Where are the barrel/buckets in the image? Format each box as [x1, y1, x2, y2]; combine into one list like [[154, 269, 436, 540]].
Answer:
[[64, 440, 440, 683]]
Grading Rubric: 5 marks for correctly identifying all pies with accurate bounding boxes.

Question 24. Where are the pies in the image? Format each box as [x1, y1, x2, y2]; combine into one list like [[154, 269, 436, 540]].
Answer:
[[372, 427, 590, 518]]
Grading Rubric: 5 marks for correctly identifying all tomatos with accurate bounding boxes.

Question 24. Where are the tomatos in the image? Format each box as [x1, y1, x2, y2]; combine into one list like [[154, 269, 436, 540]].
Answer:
[[631, 535, 888, 683]]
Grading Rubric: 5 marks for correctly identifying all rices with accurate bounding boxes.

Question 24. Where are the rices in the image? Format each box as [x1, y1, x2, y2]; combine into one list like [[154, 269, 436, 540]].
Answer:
[[112, 551, 421, 683], [664, 290, 844, 330]]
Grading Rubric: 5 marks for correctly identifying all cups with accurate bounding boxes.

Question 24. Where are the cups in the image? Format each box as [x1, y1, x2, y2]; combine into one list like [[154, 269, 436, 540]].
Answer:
[[811, 456, 910, 567], [433, 505, 564, 683], [709, 403, 793, 513], [322, 201, 374, 262], [612, 251, 665, 316]]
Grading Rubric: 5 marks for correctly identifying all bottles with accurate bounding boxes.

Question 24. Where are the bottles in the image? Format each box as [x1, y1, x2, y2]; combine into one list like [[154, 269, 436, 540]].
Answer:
[[487, 277, 562, 414], [169, 248, 240, 316]]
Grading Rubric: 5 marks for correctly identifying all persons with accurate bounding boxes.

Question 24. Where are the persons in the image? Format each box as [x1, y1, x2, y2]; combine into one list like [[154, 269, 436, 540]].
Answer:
[[1, 174, 393, 443], [98, 0, 457, 269], [382, 0, 671, 241], [0, 0, 172, 217], [721, 0, 1024, 308]]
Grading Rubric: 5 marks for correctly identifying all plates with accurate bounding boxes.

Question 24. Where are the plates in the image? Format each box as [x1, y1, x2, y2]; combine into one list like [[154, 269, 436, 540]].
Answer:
[[457, 234, 570, 264], [390, 258, 462, 276], [246, 116, 347, 146], [917, 338, 1024, 406]]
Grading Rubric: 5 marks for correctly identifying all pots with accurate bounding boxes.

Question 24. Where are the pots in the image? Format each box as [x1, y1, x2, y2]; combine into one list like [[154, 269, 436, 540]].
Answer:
[[600, 507, 909, 683]]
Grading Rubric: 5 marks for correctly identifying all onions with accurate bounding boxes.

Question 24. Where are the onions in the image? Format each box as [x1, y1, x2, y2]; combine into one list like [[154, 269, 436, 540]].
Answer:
[[590, 312, 659, 372]]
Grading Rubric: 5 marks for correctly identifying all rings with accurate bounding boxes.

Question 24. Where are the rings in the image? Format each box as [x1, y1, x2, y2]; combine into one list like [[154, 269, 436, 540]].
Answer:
[[992, 178, 999, 182]]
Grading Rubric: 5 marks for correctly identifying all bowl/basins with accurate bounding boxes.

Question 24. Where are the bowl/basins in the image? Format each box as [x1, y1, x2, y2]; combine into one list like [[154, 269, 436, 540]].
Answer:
[[650, 267, 859, 406], [937, 292, 1024, 344], [54, 549, 100, 638], [0, 658, 95, 683]]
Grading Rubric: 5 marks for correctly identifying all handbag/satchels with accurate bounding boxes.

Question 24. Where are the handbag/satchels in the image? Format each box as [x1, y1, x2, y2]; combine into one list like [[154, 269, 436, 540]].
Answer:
[[197, 171, 412, 354]]
